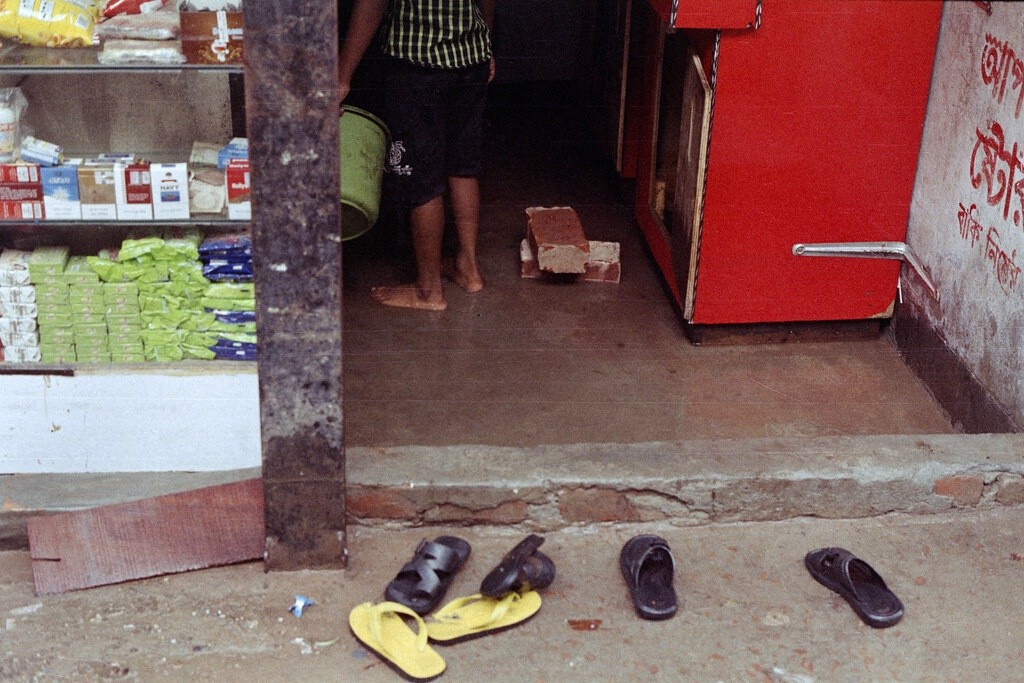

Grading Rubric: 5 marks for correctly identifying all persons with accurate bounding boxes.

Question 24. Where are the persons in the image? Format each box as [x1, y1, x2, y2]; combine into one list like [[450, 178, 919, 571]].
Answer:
[[337, 1, 495, 312]]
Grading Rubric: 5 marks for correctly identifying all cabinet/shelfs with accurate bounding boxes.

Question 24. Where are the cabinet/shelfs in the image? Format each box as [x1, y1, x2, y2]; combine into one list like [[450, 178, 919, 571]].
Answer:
[[0, 0, 257, 381]]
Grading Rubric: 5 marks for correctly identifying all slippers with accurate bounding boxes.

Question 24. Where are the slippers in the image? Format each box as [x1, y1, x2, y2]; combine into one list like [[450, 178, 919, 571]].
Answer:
[[425, 590, 542, 641], [805, 547, 905, 629], [479, 533, 556, 597], [619, 534, 678, 620], [384, 534, 472, 617], [348, 600, 446, 679]]
